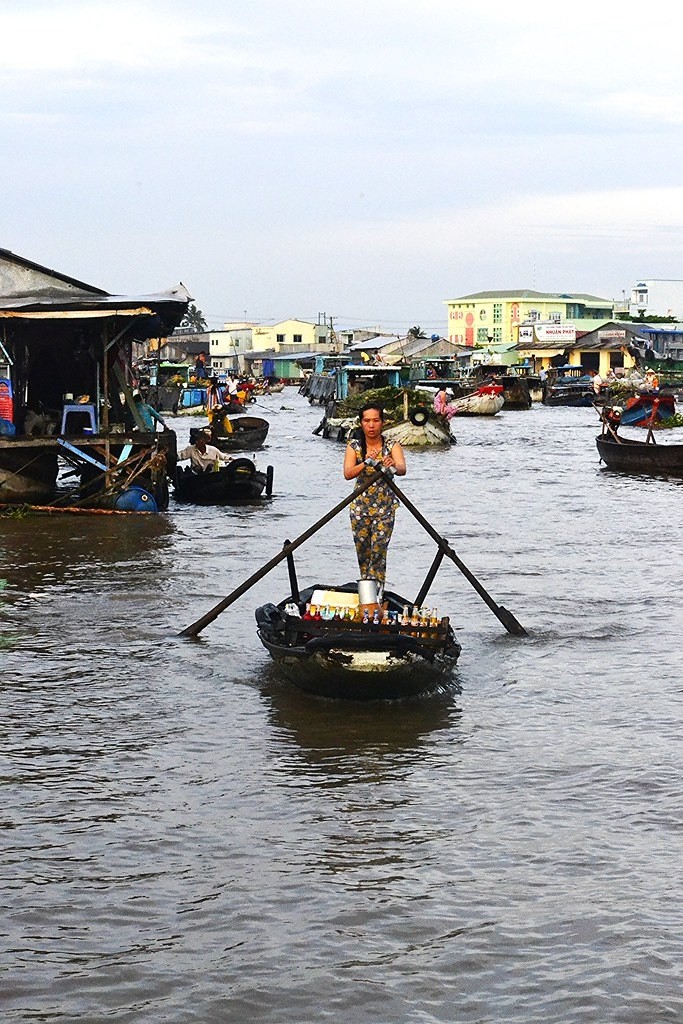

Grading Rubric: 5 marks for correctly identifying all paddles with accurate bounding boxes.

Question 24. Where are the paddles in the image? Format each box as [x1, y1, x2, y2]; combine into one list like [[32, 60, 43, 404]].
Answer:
[[363, 455, 532, 640], [175, 457, 398, 637], [238, 397, 278, 414]]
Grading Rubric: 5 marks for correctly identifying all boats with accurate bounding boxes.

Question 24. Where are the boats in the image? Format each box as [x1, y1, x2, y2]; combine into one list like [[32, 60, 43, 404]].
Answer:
[[591, 399, 683, 474], [257, 583, 464, 689], [177, 458, 273, 501], [323, 365, 458, 448], [188, 416, 270, 453], [217, 368, 284, 403], [213, 403, 246, 415], [139, 359, 207, 410], [299, 365, 676, 429]]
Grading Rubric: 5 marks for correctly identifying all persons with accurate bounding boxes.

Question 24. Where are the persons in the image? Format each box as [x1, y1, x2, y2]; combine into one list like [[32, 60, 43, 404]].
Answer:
[[344, 402, 407, 598], [262, 377, 272, 395], [538, 366, 548, 387], [487, 378, 498, 387], [206, 377, 234, 434], [588, 368, 661, 396], [133, 394, 170, 432], [176, 430, 237, 475], [426, 364, 437, 380], [194, 351, 207, 387], [433, 386, 457, 426], [238, 371, 250, 385], [547, 363, 556, 386], [223, 374, 242, 401]]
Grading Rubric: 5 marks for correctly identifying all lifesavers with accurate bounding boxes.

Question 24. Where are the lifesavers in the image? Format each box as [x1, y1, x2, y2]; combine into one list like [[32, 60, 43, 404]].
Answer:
[[409, 407, 430, 426], [225, 458, 256, 471]]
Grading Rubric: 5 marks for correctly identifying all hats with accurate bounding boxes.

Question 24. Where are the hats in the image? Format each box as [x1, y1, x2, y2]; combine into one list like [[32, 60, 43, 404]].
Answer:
[[133, 394, 142, 402], [646, 368, 655, 374]]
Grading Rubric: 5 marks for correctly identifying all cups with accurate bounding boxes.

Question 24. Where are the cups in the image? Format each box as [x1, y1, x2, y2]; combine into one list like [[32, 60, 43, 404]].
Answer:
[[356, 578, 384, 605]]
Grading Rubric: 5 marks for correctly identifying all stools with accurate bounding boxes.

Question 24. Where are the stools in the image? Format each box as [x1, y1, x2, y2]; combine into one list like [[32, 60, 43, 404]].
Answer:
[[0, 376, 14, 423], [59, 402, 98, 433]]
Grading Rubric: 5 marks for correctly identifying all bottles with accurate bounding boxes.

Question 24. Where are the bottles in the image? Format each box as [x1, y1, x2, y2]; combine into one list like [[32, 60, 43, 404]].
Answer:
[[297, 603, 438, 641], [213, 454, 221, 472]]
